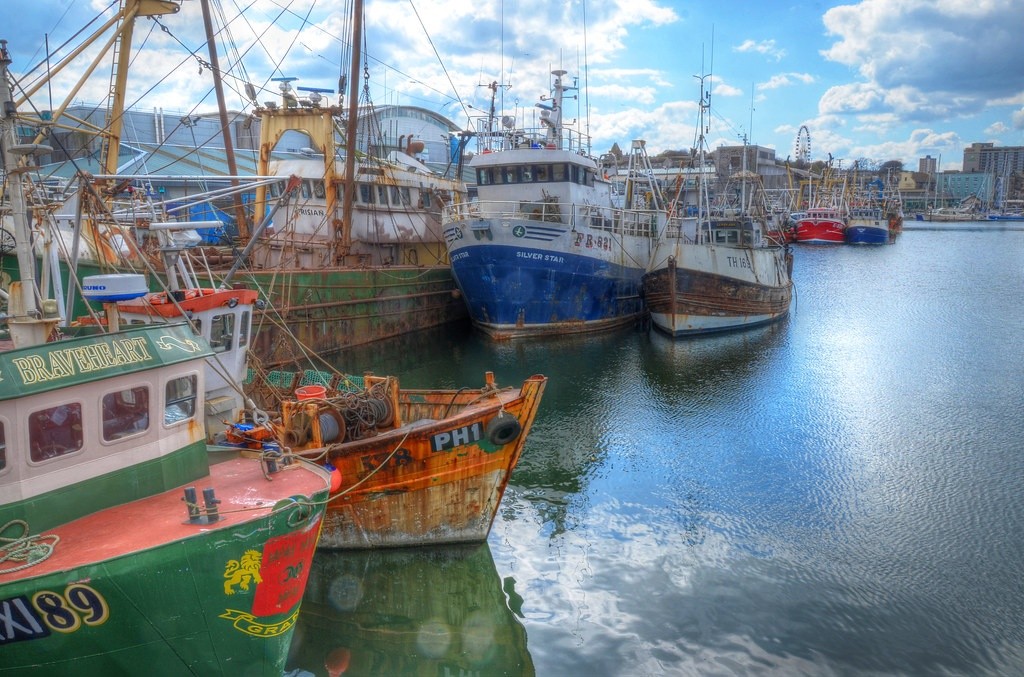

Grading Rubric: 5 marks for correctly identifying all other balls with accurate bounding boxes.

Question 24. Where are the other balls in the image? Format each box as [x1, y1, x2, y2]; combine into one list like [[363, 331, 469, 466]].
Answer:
[[545, 143, 556, 151], [531, 143, 543, 150]]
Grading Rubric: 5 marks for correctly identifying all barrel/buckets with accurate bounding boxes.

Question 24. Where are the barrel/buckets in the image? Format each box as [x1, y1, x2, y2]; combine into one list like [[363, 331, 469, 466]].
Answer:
[[295, 383, 326, 401]]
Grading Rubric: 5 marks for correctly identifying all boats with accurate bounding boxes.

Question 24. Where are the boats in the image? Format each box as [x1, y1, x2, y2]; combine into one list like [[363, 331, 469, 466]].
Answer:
[[0, 0, 1023, 676]]
